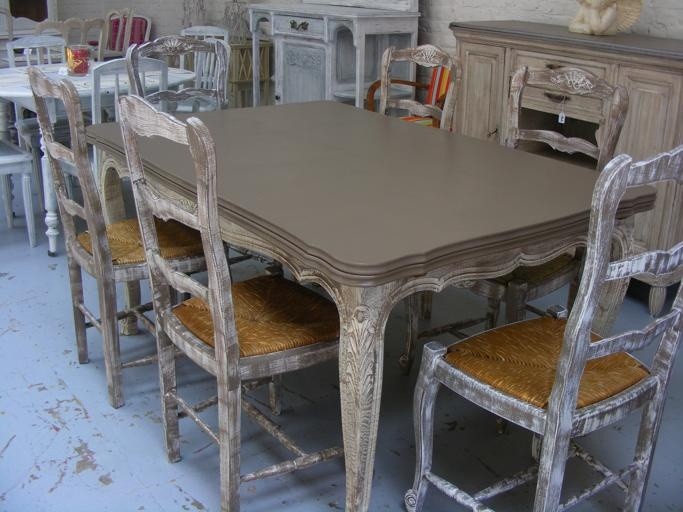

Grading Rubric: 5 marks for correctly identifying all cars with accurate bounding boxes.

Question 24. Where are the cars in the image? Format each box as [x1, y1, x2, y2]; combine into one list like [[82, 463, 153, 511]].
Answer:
[[64, 44, 96, 78]]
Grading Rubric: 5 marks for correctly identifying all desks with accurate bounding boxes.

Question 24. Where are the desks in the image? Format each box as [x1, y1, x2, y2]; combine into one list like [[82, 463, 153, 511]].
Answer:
[[450, 20, 682, 317], [247, 0, 421, 115]]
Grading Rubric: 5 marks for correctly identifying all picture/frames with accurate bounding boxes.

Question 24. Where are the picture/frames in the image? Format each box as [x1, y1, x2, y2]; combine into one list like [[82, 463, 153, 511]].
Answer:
[[450, 20, 682, 317], [247, 0, 421, 115]]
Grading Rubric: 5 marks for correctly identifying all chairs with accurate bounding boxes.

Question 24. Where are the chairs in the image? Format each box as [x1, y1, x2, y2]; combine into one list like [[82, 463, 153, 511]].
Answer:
[[403, 147, 683, 511], [0, 10, 233, 258], [113, 92, 343, 511], [374, 45, 465, 130], [398, 66, 627, 377], [26, 67, 281, 411]]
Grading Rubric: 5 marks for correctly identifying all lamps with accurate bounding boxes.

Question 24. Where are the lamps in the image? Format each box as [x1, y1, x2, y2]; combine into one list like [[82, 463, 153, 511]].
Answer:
[[113, 92, 343, 511], [374, 45, 465, 130], [403, 147, 683, 511], [26, 67, 281, 411], [398, 66, 627, 377]]
